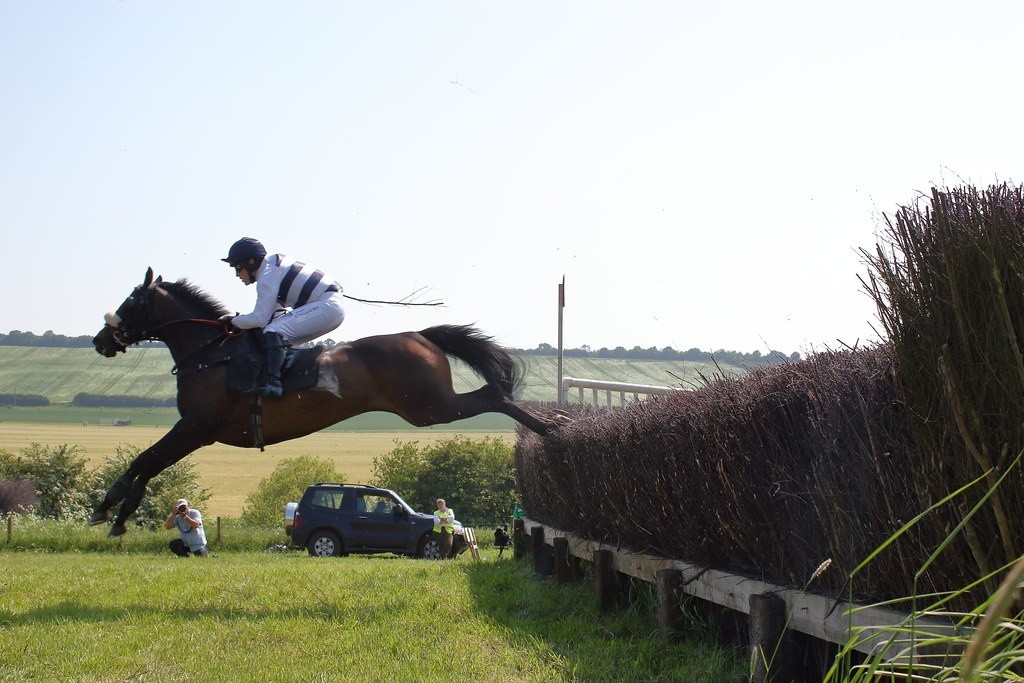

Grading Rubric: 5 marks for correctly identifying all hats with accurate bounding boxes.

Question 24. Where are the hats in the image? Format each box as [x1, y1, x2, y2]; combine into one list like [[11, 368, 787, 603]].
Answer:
[[178, 499, 187, 508]]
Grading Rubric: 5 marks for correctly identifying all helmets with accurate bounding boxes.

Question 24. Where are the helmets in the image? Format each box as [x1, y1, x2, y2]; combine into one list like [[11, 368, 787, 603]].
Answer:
[[221, 237, 267, 267]]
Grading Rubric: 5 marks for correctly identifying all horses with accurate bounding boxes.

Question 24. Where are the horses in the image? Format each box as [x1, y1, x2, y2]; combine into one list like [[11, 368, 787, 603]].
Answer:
[[88, 265, 573, 539]]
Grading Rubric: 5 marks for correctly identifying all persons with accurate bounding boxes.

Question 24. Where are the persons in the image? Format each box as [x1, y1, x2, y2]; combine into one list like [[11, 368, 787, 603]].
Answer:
[[166, 499, 208, 558], [374, 502, 386, 513], [433, 499, 454, 561], [498, 525, 509, 558], [218, 237, 345, 405]]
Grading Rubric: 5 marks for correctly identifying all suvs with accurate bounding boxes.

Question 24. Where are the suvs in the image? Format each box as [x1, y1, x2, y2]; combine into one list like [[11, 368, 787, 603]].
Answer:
[[284, 482, 469, 561]]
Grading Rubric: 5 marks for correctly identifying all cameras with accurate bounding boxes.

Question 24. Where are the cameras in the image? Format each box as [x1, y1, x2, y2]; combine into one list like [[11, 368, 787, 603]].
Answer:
[[176, 506, 186, 515]]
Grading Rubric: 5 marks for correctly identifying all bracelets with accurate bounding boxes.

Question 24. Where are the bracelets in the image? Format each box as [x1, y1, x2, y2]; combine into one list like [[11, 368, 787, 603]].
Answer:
[[183, 515, 188, 519]]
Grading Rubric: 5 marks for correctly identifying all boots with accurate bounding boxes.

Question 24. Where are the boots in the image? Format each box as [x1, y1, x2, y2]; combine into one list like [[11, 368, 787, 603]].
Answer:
[[261, 332, 285, 397]]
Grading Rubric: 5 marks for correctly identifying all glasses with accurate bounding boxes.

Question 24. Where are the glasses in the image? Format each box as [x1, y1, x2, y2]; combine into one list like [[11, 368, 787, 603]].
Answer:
[[235, 265, 243, 272]]
